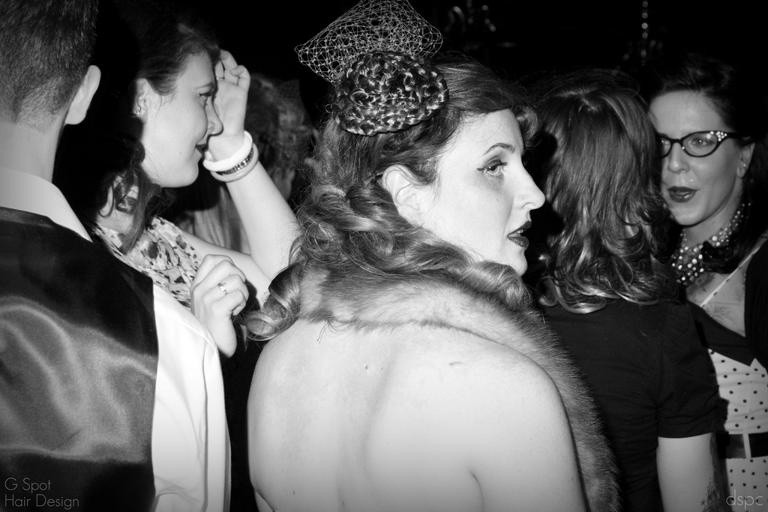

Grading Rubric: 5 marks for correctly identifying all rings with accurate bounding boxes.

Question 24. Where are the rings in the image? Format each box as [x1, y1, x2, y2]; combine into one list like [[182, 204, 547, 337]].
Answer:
[[216, 281, 229, 302]]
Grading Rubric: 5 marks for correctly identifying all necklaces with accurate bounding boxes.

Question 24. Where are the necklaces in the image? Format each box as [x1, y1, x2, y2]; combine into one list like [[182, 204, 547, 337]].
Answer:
[[661, 205, 756, 288]]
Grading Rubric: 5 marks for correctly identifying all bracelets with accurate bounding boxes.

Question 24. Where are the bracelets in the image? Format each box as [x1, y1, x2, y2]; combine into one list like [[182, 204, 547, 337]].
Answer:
[[202, 132, 258, 182]]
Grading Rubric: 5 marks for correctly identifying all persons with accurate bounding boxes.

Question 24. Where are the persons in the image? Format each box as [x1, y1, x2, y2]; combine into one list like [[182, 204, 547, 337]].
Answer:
[[0, 1, 231, 508], [649, 70, 768, 512], [518, 75, 730, 510], [242, 0, 617, 508], [61, 13, 304, 354]]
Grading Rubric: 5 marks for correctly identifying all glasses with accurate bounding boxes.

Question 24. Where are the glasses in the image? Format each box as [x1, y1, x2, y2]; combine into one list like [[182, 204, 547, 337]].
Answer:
[[656, 129, 735, 157]]
